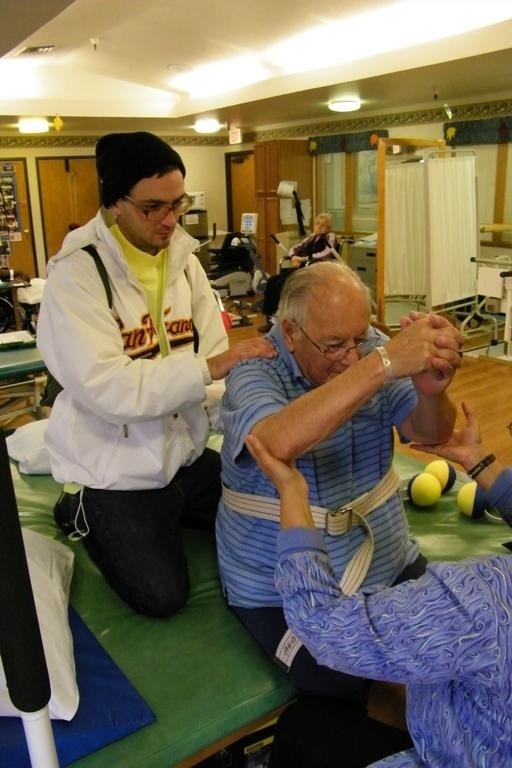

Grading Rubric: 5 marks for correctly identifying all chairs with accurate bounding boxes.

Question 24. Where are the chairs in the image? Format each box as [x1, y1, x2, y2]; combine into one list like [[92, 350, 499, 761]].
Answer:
[[279, 239, 354, 272], [206, 232, 256, 328]]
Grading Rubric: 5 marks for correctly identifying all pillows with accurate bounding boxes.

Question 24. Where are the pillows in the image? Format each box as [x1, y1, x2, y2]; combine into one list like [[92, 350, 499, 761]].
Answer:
[[0, 527, 80, 723], [1, 604, 156, 767]]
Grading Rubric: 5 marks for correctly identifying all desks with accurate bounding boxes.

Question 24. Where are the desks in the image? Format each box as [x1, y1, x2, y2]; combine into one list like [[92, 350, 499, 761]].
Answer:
[[0, 279, 31, 330]]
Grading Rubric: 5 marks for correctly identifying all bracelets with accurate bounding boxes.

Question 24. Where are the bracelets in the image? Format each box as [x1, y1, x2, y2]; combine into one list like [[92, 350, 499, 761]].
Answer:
[[372, 344, 395, 389], [465, 454, 496, 480]]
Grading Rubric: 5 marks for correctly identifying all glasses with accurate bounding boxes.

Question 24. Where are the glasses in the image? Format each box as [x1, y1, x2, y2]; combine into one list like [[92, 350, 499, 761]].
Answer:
[[290, 316, 382, 364], [122, 192, 195, 224]]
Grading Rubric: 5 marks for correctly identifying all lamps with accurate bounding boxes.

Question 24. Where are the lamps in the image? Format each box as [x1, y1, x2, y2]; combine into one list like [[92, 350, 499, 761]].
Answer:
[[327, 96, 363, 114]]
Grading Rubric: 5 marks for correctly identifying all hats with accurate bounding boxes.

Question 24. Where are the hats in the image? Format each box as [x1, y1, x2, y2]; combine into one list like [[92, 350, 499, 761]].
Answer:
[[94, 130, 188, 209]]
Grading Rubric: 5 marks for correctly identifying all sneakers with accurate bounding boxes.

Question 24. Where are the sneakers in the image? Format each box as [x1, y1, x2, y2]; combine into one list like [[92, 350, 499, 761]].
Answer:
[[256, 321, 274, 333]]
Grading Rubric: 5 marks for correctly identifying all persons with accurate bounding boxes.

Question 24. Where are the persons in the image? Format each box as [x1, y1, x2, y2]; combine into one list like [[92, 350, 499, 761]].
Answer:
[[255, 212, 337, 332], [36, 132, 279, 621], [212, 263, 465, 768], [242, 401, 511, 768]]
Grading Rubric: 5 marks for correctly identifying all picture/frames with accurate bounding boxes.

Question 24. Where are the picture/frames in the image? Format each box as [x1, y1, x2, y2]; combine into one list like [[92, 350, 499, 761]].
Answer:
[[240, 212, 258, 235], [0, 161, 16, 175]]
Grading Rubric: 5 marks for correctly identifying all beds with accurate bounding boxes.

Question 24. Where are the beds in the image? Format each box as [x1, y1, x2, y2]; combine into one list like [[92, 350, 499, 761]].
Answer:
[[0, 330, 46, 422], [1, 434, 512, 767]]
